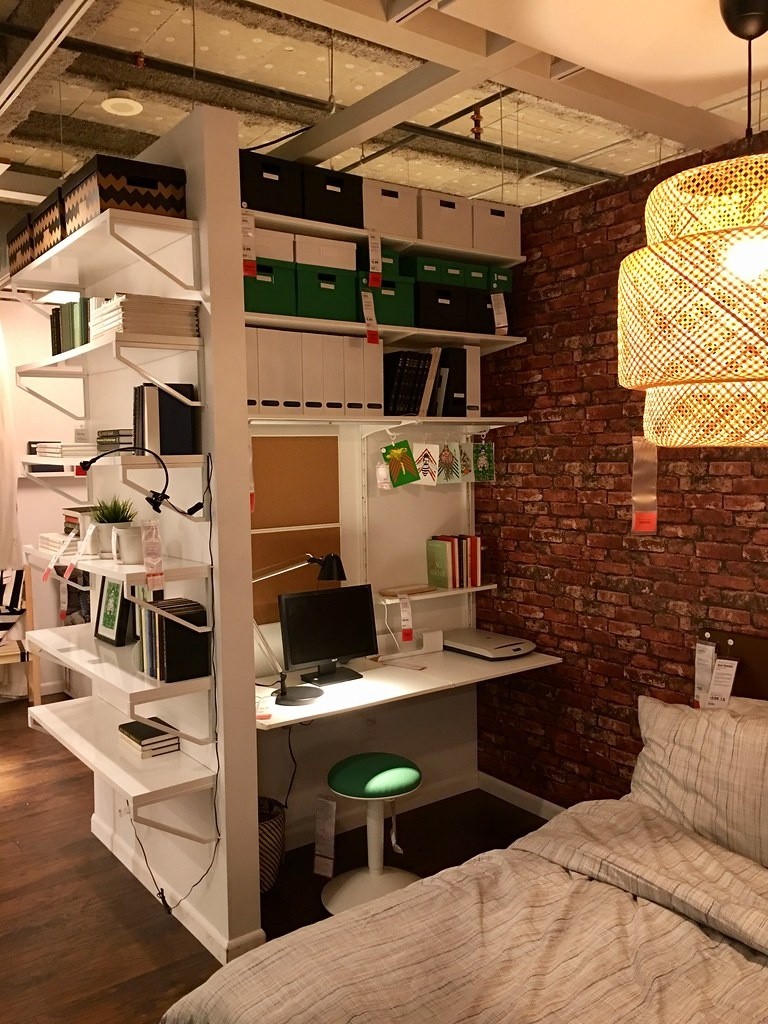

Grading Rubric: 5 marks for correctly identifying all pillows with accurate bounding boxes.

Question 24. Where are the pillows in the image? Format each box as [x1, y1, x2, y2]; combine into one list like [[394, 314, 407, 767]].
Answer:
[[624, 691, 768, 864]]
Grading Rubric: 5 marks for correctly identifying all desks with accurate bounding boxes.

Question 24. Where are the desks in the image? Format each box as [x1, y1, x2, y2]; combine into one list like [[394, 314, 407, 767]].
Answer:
[[254, 648, 564, 733]]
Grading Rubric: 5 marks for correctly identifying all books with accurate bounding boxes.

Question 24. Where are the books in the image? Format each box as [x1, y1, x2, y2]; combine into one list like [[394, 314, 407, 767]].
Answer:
[[38, 507, 107, 555], [132, 383, 195, 456], [36, 443, 97, 458], [383, 345, 451, 417], [50, 292, 202, 356], [95, 429, 134, 456], [138, 597, 210, 681], [426, 534, 481, 588], [117, 716, 180, 758]]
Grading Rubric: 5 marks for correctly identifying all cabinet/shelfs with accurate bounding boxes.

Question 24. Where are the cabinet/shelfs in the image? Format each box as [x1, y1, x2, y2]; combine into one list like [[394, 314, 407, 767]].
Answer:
[[246, 199, 530, 442], [5, 209, 219, 844]]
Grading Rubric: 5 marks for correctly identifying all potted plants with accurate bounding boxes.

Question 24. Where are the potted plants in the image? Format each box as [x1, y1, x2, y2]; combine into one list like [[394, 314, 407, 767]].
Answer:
[[88, 492, 139, 561]]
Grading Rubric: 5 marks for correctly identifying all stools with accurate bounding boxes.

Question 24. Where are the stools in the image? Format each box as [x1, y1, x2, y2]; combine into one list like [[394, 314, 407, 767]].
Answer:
[[320, 751, 425, 914]]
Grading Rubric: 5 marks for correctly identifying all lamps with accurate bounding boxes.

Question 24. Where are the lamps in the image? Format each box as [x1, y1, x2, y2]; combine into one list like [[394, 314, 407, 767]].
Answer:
[[615, 0, 768, 448], [252, 549, 347, 705]]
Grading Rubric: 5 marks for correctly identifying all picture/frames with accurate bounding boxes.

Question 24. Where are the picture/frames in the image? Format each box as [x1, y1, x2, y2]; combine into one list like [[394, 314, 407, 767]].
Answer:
[[93, 576, 133, 647]]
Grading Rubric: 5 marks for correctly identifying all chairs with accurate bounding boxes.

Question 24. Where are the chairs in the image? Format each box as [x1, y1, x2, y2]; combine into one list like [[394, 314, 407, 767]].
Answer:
[[0, 564, 42, 707]]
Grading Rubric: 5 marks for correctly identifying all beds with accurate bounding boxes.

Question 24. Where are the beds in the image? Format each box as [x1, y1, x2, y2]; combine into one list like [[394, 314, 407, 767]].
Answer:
[[160, 625, 768, 1024]]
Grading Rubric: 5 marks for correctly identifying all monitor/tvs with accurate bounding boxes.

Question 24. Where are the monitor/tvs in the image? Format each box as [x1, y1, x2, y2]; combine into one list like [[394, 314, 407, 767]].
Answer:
[[278, 584, 379, 687]]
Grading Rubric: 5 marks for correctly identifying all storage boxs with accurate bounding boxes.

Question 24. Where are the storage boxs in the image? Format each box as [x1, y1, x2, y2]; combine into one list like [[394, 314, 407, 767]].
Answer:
[[6, 153, 188, 280], [380, 247, 402, 274], [296, 262, 359, 320], [417, 282, 471, 330], [469, 289, 509, 335], [239, 148, 302, 218], [473, 196, 524, 254], [253, 226, 294, 261], [302, 163, 362, 227], [413, 256, 440, 280], [295, 231, 357, 271], [419, 186, 472, 250], [248, 327, 482, 420], [362, 175, 418, 239], [465, 264, 489, 288], [440, 260, 465, 285], [490, 266, 514, 290], [359, 268, 416, 323], [244, 256, 297, 316]]
